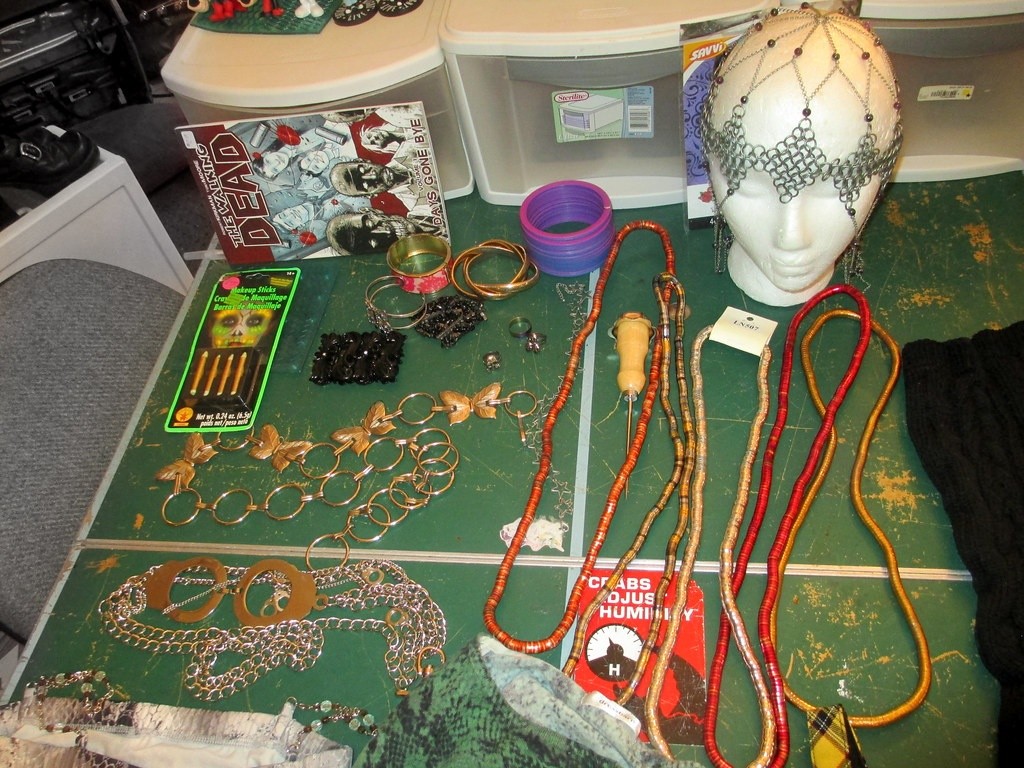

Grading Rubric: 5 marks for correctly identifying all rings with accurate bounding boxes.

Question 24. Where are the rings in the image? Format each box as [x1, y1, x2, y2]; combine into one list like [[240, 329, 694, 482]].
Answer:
[[508, 316, 533, 337]]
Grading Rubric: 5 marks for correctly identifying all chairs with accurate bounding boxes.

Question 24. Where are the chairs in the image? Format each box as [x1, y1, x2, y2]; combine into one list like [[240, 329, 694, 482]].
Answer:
[[0, 259, 185, 636]]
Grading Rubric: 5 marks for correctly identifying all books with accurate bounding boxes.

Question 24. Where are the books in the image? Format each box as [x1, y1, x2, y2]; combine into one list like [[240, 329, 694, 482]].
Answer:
[[173, 101, 451, 266]]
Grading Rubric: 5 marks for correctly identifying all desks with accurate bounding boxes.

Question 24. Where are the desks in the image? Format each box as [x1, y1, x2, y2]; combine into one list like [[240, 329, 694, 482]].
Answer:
[[0, 143, 193, 296], [0, 171, 1024, 768]]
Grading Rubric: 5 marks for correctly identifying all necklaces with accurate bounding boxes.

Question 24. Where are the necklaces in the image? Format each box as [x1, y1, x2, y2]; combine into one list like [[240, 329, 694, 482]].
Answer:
[[412, 296, 486, 348], [485, 219, 933, 768]]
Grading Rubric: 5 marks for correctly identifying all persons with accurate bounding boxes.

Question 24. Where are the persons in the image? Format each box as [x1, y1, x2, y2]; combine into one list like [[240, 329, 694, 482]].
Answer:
[[702, 9, 904, 307]]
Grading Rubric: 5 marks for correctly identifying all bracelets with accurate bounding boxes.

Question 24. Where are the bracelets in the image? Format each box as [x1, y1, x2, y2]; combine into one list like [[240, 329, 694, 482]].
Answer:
[[360, 180, 614, 333]]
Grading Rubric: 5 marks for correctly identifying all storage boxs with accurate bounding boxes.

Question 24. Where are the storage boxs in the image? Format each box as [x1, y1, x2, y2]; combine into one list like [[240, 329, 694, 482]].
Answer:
[[160, 0, 474, 201], [860, 0, 1024, 183], [440, 1, 779, 211]]
